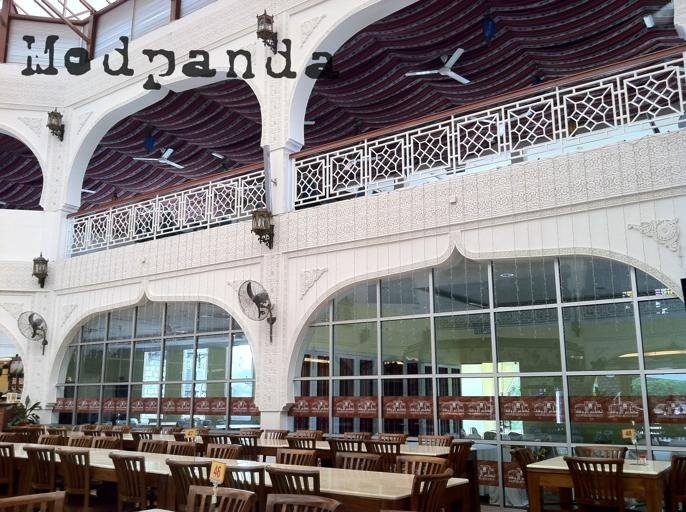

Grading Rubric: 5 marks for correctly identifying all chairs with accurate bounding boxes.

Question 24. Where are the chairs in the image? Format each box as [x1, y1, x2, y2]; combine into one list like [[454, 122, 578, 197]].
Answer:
[[509, 442, 686, 512], [0, 422, 482, 512]]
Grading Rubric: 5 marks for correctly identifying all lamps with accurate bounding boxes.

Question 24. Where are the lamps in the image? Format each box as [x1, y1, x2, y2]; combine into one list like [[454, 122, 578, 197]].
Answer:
[[31, 249, 50, 288], [250, 200, 275, 250], [46, 104, 66, 143], [255, 6, 277, 56]]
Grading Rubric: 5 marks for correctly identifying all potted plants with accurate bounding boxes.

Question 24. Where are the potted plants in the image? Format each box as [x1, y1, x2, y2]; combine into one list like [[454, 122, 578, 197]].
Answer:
[[6, 392, 44, 430]]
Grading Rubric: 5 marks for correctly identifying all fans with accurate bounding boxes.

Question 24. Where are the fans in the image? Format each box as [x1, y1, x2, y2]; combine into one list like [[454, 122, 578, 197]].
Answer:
[[404, 46, 472, 85], [238, 278, 276, 344], [132, 148, 185, 172], [18, 311, 48, 355]]
